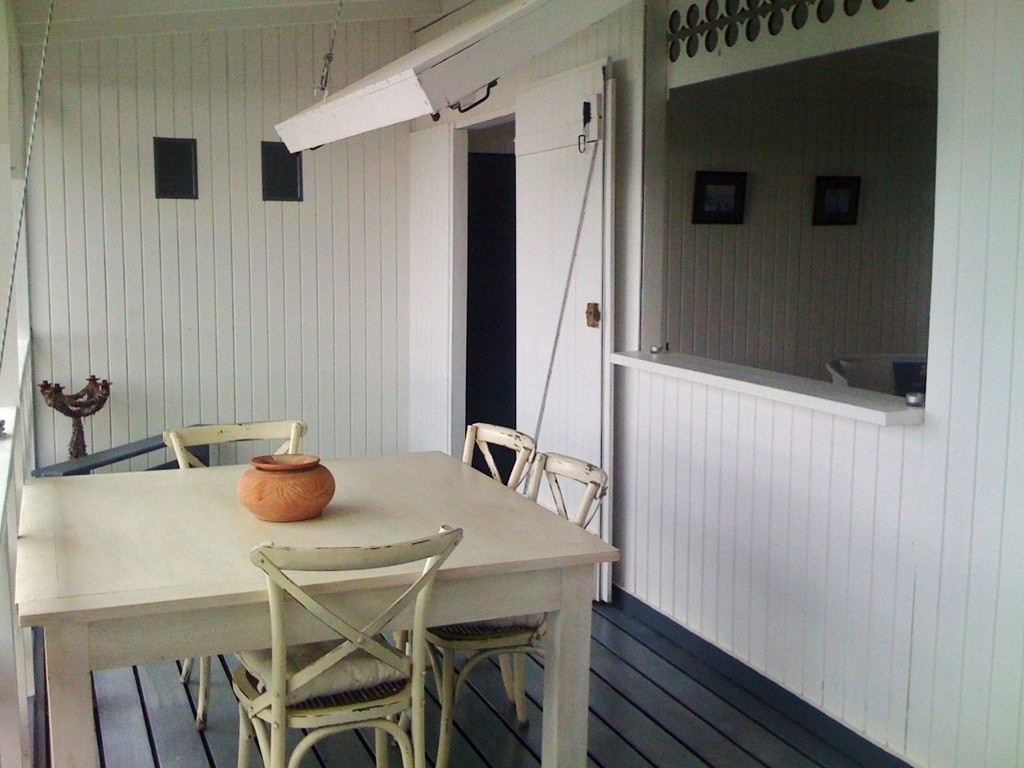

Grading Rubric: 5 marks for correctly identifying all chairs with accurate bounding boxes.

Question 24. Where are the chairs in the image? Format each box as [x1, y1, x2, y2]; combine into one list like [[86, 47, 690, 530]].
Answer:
[[161, 421, 609, 766]]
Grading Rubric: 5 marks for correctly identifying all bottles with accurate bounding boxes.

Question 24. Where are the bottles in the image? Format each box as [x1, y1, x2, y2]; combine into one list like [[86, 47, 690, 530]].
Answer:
[[238, 454, 336, 522]]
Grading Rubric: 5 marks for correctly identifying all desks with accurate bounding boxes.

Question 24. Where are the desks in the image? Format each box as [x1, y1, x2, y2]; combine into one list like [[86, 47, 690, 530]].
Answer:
[[13, 452, 618, 766]]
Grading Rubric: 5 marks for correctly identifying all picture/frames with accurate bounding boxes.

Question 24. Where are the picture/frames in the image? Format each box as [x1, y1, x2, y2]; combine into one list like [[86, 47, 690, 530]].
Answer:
[[813, 176, 862, 225], [690, 171, 749, 224]]
